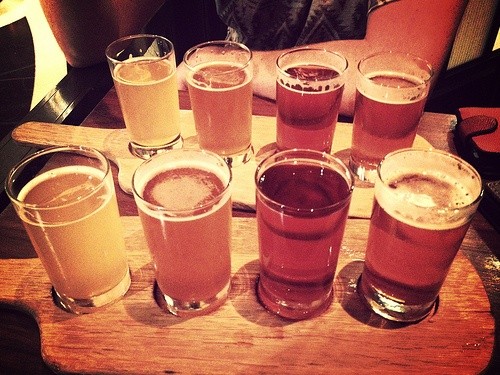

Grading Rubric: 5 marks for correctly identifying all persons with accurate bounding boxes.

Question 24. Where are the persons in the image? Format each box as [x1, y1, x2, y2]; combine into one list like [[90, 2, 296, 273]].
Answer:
[[38, 0, 468, 121]]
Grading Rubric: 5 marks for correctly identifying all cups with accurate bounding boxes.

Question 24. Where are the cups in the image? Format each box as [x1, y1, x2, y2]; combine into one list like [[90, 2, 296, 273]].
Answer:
[[182, 40, 253, 166], [253, 148, 353, 321], [131, 147, 233, 317], [2, 146, 131, 313], [105, 33, 184, 157], [358, 148, 484, 322], [351, 51, 434, 177], [275, 49, 349, 164]]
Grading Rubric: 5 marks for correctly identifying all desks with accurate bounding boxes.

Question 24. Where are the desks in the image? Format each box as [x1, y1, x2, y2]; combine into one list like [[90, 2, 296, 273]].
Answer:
[[0, 67, 500, 375]]
